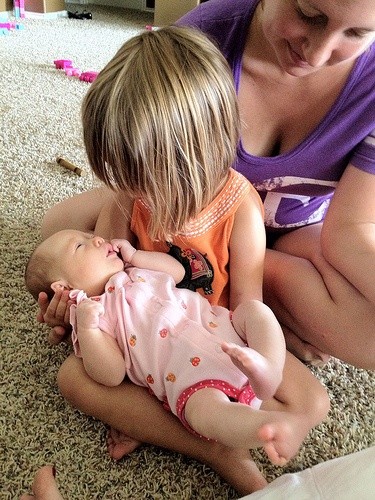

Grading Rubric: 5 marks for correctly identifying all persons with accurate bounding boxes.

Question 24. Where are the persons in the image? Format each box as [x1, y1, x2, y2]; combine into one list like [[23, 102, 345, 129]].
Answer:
[[42, 1, 375, 373], [12, 466, 67, 500], [44, 27, 330, 495], [25, 229, 310, 468]]
